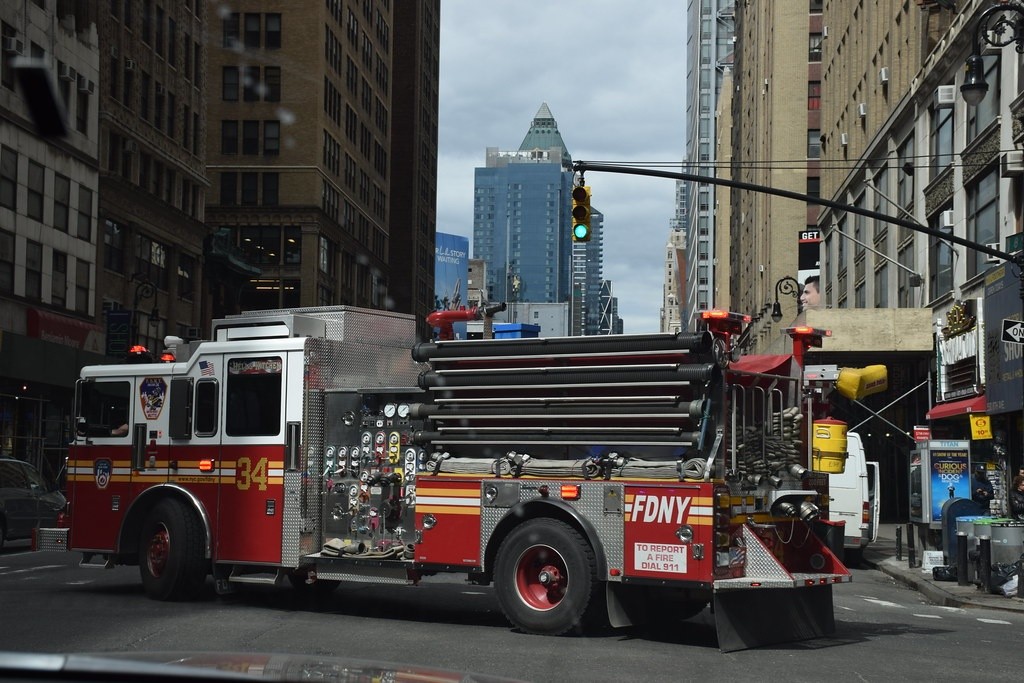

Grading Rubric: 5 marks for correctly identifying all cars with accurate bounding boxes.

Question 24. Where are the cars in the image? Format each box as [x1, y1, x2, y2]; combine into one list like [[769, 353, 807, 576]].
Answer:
[[0, 458, 67, 552]]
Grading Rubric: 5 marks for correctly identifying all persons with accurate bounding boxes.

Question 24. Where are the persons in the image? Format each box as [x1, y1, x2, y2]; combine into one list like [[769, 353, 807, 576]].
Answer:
[[800, 276, 819, 311], [112, 421, 128, 435], [1009, 466, 1024, 514], [972, 465, 994, 508]]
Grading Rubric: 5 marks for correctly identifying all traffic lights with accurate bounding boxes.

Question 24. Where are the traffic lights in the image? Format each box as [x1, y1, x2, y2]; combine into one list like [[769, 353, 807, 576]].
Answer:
[[571, 187, 592, 242]]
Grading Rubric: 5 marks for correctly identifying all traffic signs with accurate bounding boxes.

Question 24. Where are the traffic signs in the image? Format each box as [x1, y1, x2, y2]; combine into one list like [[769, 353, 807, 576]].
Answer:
[[1001, 319, 1024, 344]]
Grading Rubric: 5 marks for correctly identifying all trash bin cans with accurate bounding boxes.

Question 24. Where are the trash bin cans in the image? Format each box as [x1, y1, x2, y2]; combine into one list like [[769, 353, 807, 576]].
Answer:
[[991, 522, 1024, 576], [941, 498, 980, 566], [956, 515, 1015, 582]]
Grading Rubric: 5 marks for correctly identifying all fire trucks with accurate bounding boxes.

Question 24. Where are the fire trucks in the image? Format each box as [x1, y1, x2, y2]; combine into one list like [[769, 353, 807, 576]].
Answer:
[[32, 302, 854, 654]]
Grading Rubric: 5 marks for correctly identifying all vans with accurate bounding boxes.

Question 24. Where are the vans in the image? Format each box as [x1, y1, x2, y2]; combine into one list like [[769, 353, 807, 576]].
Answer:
[[829, 431, 881, 568]]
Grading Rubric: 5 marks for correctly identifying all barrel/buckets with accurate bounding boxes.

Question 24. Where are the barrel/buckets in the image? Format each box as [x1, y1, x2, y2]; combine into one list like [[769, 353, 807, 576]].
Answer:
[[812, 416, 847, 473], [992, 523, 1024, 581], [974, 518, 1014, 580], [956, 516, 993, 582]]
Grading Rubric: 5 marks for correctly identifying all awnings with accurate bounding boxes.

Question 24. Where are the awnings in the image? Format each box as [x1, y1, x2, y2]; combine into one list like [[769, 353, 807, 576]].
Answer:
[[926, 394, 987, 419]]
